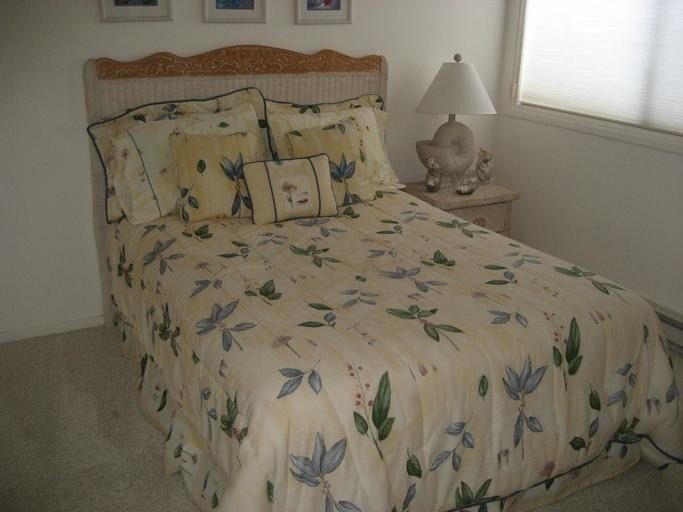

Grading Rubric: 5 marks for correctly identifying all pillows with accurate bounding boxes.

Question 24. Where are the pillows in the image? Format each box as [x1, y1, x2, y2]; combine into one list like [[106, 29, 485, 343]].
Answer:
[[262, 94, 406, 190], [241, 153, 336, 227], [168, 129, 267, 227], [282, 114, 384, 206], [86, 87, 274, 226]]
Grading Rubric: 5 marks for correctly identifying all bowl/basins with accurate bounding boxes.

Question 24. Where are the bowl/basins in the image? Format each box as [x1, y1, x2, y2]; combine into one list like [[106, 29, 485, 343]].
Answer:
[[453, 174, 478, 194]]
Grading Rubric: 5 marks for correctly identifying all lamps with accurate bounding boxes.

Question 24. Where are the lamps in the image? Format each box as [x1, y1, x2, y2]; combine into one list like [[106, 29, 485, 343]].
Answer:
[[414, 53, 498, 195]]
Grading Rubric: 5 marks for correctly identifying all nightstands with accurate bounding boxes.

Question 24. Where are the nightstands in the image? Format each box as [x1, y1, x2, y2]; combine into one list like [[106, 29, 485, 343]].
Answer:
[[406, 176, 520, 240]]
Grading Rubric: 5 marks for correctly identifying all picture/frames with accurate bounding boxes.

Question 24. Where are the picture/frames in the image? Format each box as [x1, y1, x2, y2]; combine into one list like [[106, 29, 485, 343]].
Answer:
[[97, 0, 352, 26]]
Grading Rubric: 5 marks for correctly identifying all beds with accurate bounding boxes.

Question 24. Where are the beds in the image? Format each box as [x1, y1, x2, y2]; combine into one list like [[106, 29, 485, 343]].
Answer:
[[81, 45, 663, 512]]
